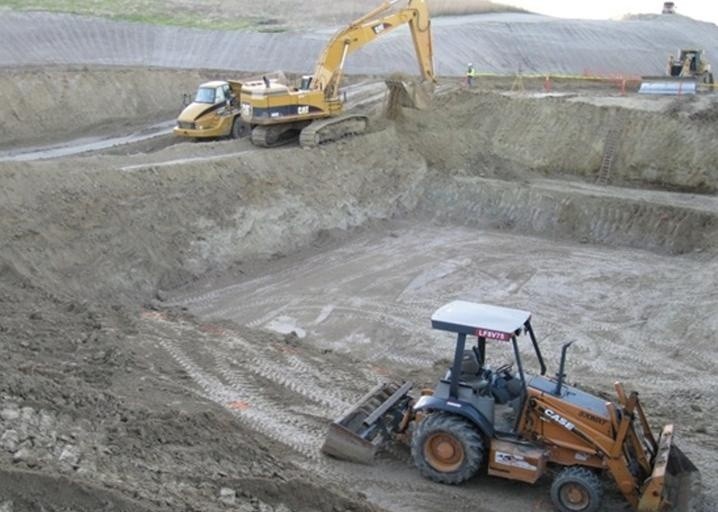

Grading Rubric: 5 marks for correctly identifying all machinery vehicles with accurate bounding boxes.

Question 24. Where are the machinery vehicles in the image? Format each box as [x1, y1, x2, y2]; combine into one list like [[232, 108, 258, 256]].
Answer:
[[637, 49, 713, 97], [320, 299, 702, 512], [239, 1, 437, 148], [172, 71, 294, 141]]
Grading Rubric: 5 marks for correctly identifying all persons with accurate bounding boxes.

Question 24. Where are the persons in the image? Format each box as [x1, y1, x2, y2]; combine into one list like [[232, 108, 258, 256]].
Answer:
[[666, 55, 675, 76], [465, 63, 475, 87]]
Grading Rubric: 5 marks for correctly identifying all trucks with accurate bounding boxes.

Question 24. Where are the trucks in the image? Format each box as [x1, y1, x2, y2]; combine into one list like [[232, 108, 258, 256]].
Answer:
[[661, 2, 676, 15]]
